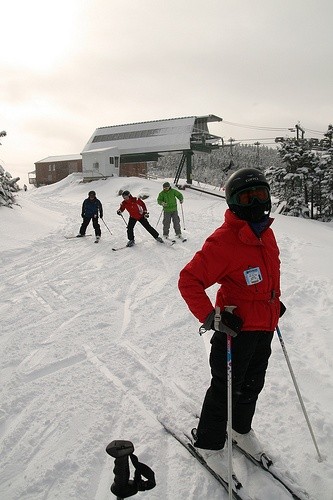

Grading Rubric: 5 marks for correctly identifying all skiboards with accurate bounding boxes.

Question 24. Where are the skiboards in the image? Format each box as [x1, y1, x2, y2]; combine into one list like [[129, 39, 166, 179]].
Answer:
[[165, 234, 188, 244], [183, 407, 319, 500], [112, 238, 187, 252], [153, 407, 259, 500], [64, 234, 100, 244]]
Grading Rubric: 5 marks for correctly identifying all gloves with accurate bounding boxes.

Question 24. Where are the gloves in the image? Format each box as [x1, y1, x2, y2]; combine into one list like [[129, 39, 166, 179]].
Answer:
[[203, 309, 242, 338], [180, 200, 183, 204], [162, 202, 167, 208], [81, 212, 84, 217], [117, 209, 121, 215], [143, 212, 149, 217], [279, 301, 287, 319], [100, 214, 102, 218]]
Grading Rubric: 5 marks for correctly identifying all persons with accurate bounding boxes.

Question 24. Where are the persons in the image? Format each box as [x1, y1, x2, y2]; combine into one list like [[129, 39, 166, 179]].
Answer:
[[117, 191, 162, 247], [157, 182, 183, 236], [77, 191, 103, 239], [178, 167, 286, 463]]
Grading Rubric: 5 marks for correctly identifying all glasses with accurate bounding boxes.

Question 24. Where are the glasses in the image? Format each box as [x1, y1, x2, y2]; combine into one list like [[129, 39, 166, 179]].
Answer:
[[233, 186, 270, 206]]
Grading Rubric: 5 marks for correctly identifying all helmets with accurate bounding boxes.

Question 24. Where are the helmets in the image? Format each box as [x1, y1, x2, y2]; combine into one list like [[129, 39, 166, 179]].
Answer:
[[162, 182, 170, 187], [226, 168, 271, 223], [122, 191, 131, 197], [88, 191, 96, 199]]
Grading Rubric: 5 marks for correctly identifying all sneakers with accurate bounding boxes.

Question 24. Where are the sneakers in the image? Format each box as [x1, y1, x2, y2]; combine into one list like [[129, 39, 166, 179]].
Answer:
[[126, 240, 134, 247], [96, 236, 100, 241], [156, 237, 163, 243], [177, 234, 182, 239], [76, 234, 85, 237], [164, 235, 169, 240]]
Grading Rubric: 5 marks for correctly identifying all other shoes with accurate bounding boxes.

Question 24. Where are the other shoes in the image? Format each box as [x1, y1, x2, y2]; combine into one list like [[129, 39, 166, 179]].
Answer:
[[197, 448, 234, 479], [227, 427, 263, 455]]
